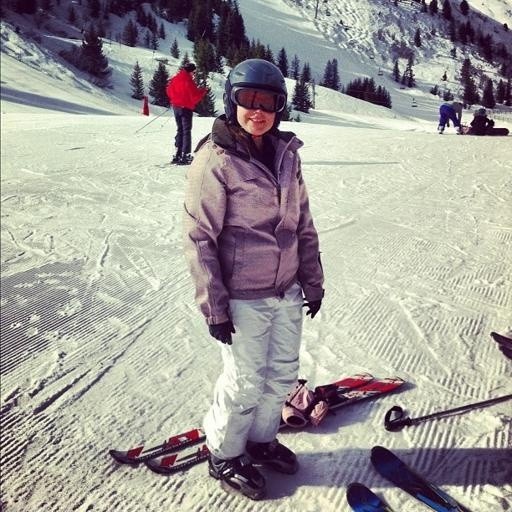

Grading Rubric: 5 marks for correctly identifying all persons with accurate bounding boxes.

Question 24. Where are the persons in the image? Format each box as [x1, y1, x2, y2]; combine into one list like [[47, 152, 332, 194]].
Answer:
[[437, 101, 467, 135], [179, 56, 325, 503], [470, 108, 494, 132], [165, 62, 209, 165]]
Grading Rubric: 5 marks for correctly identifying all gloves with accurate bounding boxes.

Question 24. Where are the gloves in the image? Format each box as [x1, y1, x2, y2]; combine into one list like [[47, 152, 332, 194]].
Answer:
[[209, 320, 236, 345], [303, 297, 321, 318]]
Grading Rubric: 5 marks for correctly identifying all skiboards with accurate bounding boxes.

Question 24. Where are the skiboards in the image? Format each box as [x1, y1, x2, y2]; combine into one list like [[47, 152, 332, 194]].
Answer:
[[347, 446, 471, 512], [108, 372, 406, 472], [153, 159, 195, 168]]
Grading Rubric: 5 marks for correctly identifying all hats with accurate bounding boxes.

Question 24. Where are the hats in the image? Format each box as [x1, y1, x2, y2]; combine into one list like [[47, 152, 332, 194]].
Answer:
[[184, 63, 196, 72]]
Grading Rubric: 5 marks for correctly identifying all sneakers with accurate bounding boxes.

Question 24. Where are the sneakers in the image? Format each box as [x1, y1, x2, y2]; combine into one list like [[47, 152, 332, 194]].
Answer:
[[207, 450, 266, 500], [176, 152, 193, 161], [244, 438, 299, 474]]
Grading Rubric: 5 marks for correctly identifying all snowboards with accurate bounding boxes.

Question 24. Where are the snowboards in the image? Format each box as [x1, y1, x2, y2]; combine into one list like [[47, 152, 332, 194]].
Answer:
[[456, 126, 509, 136]]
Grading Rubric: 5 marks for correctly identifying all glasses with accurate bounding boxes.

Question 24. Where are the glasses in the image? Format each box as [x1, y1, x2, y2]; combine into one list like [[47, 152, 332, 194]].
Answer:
[[225, 78, 286, 113]]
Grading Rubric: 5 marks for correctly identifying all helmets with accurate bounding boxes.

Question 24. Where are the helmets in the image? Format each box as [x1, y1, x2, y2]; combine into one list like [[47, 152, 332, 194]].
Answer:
[[223, 59, 286, 118]]
